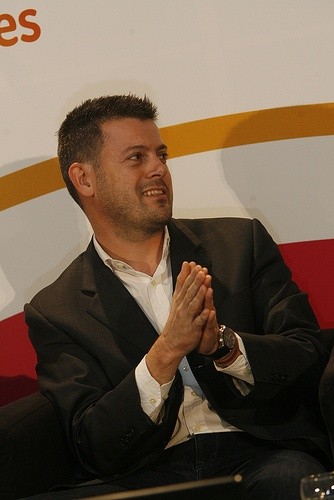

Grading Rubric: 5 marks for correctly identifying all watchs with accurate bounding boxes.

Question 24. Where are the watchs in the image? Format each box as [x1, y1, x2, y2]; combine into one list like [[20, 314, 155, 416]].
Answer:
[[200, 324, 237, 361]]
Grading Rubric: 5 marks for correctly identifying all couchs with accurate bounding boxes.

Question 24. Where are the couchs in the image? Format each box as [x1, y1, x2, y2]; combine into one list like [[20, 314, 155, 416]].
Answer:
[[0, 330, 334, 500]]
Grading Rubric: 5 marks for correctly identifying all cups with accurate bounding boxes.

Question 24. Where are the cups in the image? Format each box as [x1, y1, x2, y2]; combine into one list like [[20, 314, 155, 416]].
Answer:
[[300, 473, 334, 500]]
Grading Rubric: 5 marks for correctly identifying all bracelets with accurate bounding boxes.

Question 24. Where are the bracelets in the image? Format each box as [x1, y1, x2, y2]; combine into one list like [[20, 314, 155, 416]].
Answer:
[[212, 344, 239, 365]]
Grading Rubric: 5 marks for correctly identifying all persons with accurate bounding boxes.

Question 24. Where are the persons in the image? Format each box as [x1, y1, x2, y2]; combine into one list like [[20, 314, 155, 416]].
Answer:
[[23, 94, 332, 500]]
